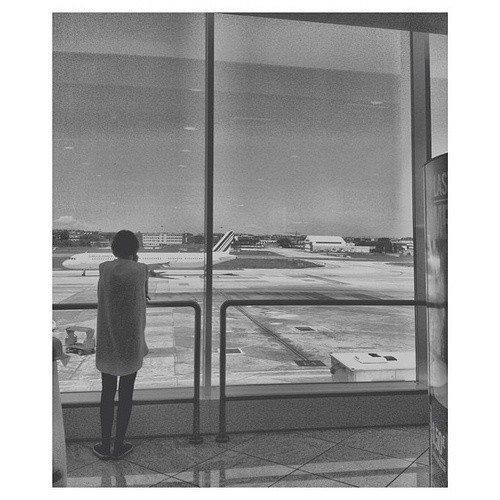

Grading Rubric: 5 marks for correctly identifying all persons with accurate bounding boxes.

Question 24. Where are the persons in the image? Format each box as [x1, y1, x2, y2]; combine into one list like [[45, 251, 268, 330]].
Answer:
[[92, 230, 148, 461]]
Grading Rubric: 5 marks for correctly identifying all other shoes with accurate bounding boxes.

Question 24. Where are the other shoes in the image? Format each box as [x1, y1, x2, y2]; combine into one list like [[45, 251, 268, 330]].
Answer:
[[92, 443, 110, 460], [112, 442, 133, 459]]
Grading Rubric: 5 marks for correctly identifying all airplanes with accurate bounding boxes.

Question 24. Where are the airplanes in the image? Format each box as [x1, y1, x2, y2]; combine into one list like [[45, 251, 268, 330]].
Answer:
[[62, 231, 237, 277]]
